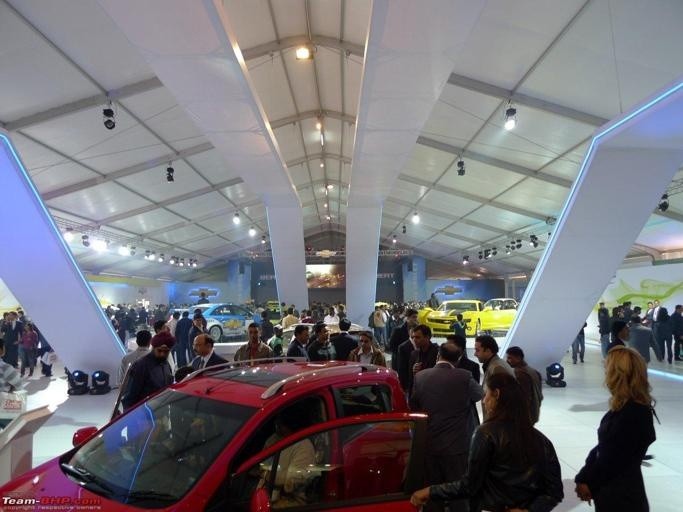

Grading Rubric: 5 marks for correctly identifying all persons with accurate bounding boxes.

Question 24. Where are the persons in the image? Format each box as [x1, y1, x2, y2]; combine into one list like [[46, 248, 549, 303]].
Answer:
[[333, 302, 337, 314], [429, 294, 441, 311], [324, 306, 339, 325], [256, 304, 265, 314], [656, 307, 673, 365], [187, 314, 214, 362], [305, 320, 328, 349], [174, 311, 194, 369], [166, 312, 184, 365], [151, 320, 177, 361], [449, 315, 469, 359], [183, 333, 230, 372], [412, 323, 436, 372], [388, 309, 419, 370], [121, 332, 174, 409], [260, 311, 273, 342], [506, 345, 544, 426], [409, 372, 564, 512], [116, 328, 152, 393], [445, 333, 482, 423], [328, 317, 358, 364], [230, 409, 323, 512], [373, 301, 427, 313], [166, 300, 194, 310], [308, 323, 337, 362], [643, 303, 652, 326], [652, 300, 661, 353], [278, 307, 300, 329], [671, 304, 683, 363], [198, 291, 210, 302], [286, 325, 312, 362], [571, 320, 587, 363], [231, 323, 276, 368], [398, 326, 412, 393], [338, 306, 351, 322], [193, 309, 207, 330], [279, 302, 288, 315], [367, 306, 383, 346], [299, 297, 330, 320], [348, 331, 387, 369], [613, 301, 630, 320], [339, 300, 346, 308], [597, 302, 612, 356], [289, 304, 297, 317], [631, 307, 643, 322], [574, 343, 661, 512], [242, 299, 255, 307], [606, 322, 655, 466], [0, 306, 56, 377], [473, 335, 517, 421], [374, 307, 387, 349], [105, 303, 165, 336], [407, 343, 485, 485]]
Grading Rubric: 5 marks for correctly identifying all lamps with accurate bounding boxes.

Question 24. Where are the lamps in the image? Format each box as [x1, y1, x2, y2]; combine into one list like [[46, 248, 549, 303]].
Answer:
[[130, 247, 136, 255], [165, 167, 175, 182], [465, 256, 469, 263], [175, 257, 178, 266], [158, 254, 165, 263], [529, 235, 538, 248], [187, 258, 192, 266], [456, 160, 466, 177], [487, 249, 492, 258], [63, 367, 91, 395], [178, 258, 184, 267], [261, 235, 266, 244], [169, 256, 176, 265], [463, 257, 466, 265], [392, 235, 398, 243], [401, 226, 406, 233], [484, 250, 488, 259], [505, 245, 511, 255], [144, 250, 151, 260], [249, 226, 257, 238], [192, 259, 198, 267], [81, 235, 90, 246], [510, 242, 516, 250], [89, 371, 111, 396], [658, 194, 669, 213], [492, 248, 497, 256], [504, 108, 517, 129], [413, 213, 419, 223], [233, 213, 241, 224], [478, 252, 483, 259], [148, 251, 156, 261], [516, 240, 522, 248], [101, 108, 116, 130], [545, 362, 567, 389]]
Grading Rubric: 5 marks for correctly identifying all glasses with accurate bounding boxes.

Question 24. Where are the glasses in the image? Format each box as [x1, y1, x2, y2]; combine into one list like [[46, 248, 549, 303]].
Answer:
[[193, 342, 205, 349], [359, 338, 368, 343]]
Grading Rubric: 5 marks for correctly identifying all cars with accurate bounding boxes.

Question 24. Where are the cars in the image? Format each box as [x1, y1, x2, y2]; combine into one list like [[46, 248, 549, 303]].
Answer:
[[1, 356, 430, 512]]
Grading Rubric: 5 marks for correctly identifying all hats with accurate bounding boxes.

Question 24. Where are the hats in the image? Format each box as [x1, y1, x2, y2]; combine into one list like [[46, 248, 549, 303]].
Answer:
[[151, 331, 176, 348]]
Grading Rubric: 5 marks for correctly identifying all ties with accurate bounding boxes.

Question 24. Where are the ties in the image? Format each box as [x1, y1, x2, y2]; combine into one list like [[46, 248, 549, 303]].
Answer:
[[198, 357, 205, 369]]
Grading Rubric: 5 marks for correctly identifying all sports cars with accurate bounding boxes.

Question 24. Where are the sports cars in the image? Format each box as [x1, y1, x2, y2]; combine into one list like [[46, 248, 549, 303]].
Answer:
[[188, 303, 262, 343], [418, 297, 518, 337]]
